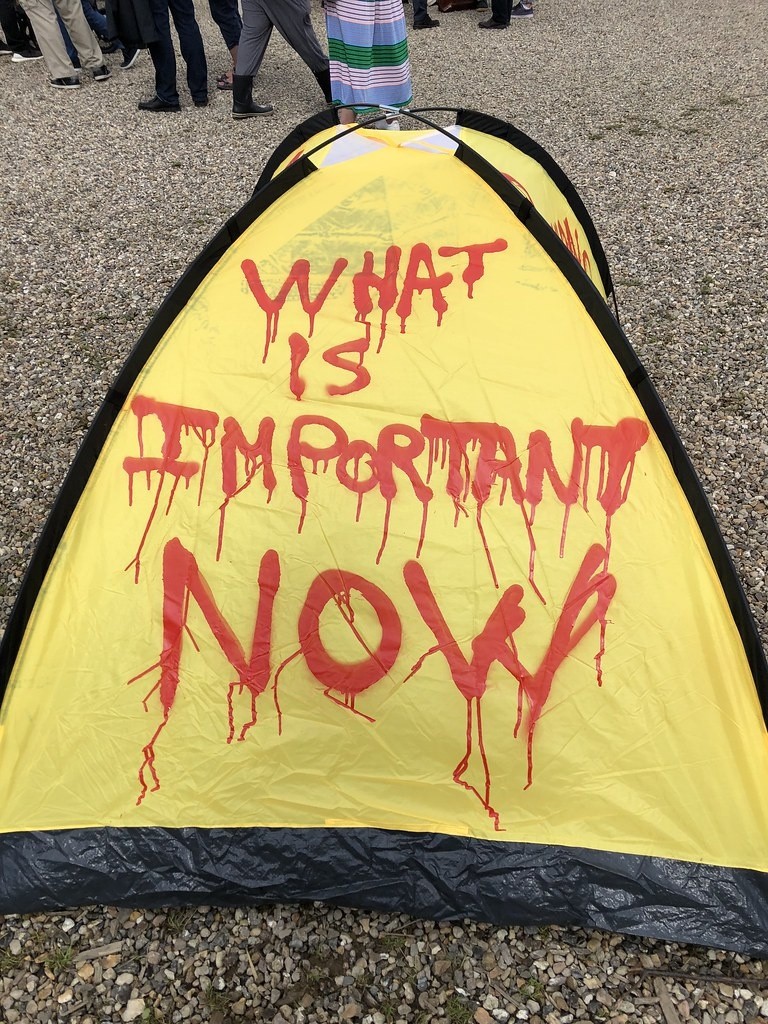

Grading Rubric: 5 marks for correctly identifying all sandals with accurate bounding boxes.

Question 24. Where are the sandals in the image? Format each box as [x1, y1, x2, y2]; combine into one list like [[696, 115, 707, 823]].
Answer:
[[217, 77, 234, 90], [217, 66, 236, 81]]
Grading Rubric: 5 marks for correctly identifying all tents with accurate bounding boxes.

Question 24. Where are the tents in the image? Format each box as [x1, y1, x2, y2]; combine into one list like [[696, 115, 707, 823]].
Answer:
[[0, 100, 768, 956]]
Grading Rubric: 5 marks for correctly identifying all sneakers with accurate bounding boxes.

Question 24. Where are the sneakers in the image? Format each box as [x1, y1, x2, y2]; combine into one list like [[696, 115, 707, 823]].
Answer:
[[0, 39, 13, 56], [511, 2, 534, 19], [71, 56, 82, 72], [94, 65, 112, 80], [119, 47, 140, 69], [10, 45, 44, 62], [374, 120, 399, 131], [478, 19, 507, 29], [48, 77, 80, 89], [413, 14, 439, 29]]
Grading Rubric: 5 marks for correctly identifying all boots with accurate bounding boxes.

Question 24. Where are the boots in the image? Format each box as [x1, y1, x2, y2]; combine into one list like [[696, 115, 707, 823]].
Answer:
[[231, 73, 274, 117], [314, 68, 332, 105]]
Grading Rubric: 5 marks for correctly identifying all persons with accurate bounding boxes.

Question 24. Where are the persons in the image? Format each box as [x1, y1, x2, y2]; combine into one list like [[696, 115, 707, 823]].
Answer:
[[477, 0, 513, 30], [0, 0, 43, 63], [511, 0, 535, 19], [209, 0, 244, 91], [412, 1, 441, 30], [19, 0, 113, 90], [113, 0, 208, 113], [54, 0, 141, 72], [232, 0, 332, 121], [325, 0, 413, 131]]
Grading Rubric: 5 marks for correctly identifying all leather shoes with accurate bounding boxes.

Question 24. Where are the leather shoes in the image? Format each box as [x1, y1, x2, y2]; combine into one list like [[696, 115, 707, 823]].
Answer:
[[137, 95, 181, 112], [196, 96, 208, 107]]
